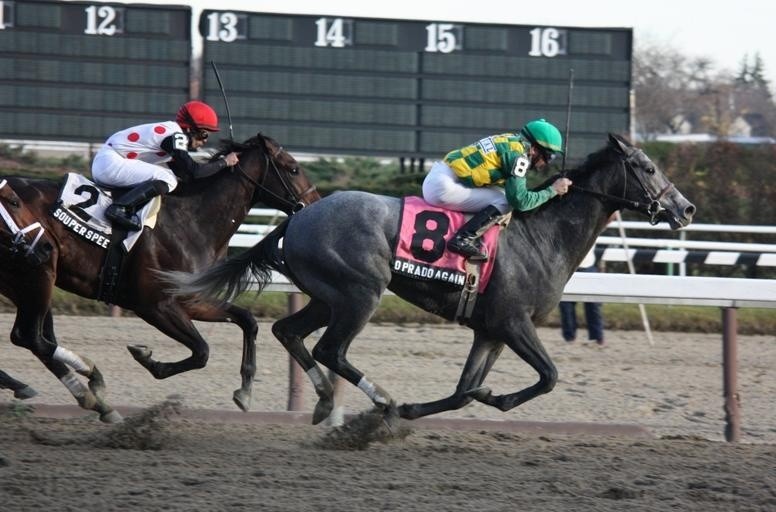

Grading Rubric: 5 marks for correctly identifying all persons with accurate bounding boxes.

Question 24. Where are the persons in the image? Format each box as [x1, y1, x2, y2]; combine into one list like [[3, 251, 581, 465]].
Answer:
[[559, 232, 607, 345], [421, 118, 572, 262], [91, 99, 243, 231]]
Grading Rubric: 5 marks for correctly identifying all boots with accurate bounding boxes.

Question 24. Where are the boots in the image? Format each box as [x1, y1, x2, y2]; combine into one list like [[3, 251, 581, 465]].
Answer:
[[104, 179, 158, 231], [447, 205, 502, 263]]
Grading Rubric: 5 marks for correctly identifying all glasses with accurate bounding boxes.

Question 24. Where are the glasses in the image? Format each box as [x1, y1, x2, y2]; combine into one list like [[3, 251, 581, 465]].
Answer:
[[524, 124, 557, 164], [180, 104, 208, 141]]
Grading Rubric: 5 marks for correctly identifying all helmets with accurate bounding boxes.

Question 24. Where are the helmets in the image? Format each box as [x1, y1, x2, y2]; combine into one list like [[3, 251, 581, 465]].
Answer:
[[520, 117, 562, 154], [175, 100, 220, 133]]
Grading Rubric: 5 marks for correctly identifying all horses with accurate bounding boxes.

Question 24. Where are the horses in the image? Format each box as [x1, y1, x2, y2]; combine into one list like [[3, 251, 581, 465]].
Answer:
[[0, 177, 56, 399], [0, 132, 322, 424], [146, 132, 696, 438]]
